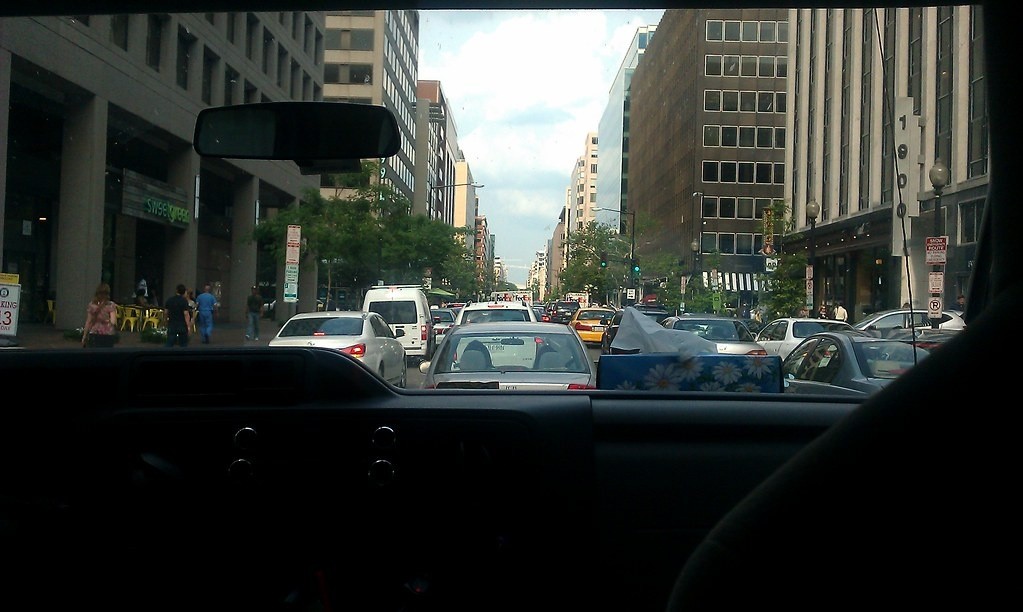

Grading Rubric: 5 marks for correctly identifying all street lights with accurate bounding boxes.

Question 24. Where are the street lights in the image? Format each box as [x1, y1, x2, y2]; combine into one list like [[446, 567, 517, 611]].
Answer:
[[693, 192, 704, 254], [929, 160, 949, 353], [692, 239, 701, 300], [429, 181, 484, 221], [806, 196, 820, 319], [591, 207, 635, 305], [543, 268, 559, 292]]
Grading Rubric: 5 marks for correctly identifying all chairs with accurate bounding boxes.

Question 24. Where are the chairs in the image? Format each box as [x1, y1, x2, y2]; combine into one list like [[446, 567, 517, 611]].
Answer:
[[538, 351, 563, 370], [107, 303, 200, 335], [460, 350, 486, 370]]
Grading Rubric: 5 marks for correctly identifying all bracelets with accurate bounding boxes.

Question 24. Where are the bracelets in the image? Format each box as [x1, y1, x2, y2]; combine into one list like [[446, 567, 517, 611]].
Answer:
[[82, 336, 87, 340]]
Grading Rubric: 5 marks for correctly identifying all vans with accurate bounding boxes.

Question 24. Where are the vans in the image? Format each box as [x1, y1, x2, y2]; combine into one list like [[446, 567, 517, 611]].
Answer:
[[362, 285, 439, 367]]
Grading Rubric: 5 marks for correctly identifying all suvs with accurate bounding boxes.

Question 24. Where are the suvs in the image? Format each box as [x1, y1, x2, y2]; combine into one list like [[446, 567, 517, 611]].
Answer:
[[442, 300, 544, 370], [600, 305, 672, 355]]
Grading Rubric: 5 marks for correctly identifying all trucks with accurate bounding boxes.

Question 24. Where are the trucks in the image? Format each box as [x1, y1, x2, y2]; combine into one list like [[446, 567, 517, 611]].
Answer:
[[490, 292, 533, 308], [566, 293, 588, 307]]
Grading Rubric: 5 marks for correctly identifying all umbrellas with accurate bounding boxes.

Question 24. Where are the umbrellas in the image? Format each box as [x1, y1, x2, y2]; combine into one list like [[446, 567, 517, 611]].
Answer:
[[426, 288, 454, 295]]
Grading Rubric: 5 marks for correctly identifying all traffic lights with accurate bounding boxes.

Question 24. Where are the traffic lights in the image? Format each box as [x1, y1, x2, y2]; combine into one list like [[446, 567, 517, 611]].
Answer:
[[600, 252, 606, 267], [635, 259, 640, 273]]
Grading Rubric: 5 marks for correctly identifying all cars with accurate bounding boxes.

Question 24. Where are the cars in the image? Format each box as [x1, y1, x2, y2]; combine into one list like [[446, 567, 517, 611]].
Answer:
[[533, 300, 581, 324], [268, 312, 407, 388], [852, 308, 967, 331], [429, 303, 466, 348], [782, 327, 931, 397], [419, 323, 597, 390], [567, 305, 616, 345], [660, 314, 767, 355], [751, 317, 857, 363]]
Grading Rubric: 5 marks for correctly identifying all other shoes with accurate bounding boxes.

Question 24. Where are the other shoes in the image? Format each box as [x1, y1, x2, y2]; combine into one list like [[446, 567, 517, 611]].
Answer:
[[204, 333, 209, 345], [245, 335, 250, 340], [255, 337, 259, 341]]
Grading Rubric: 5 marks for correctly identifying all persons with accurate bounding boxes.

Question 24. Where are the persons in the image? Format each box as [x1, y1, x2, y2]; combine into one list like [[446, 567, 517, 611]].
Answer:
[[323, 294, 335, 311], [949, 295, 964, 311], [182, 281, 220, 324], [81, 283, 117, 350], [163, 284, 190, 348], [902, 303, 915, 325], [195, 285, 218, 343], [833, 301, 847, 322], [244, 286, 263, 341], [817, 305, 828, 320], [827, 303, 837, 320], [754, 310, 762, 324], [136, 289, 145, 306], [137, 274, 147, 298]]
